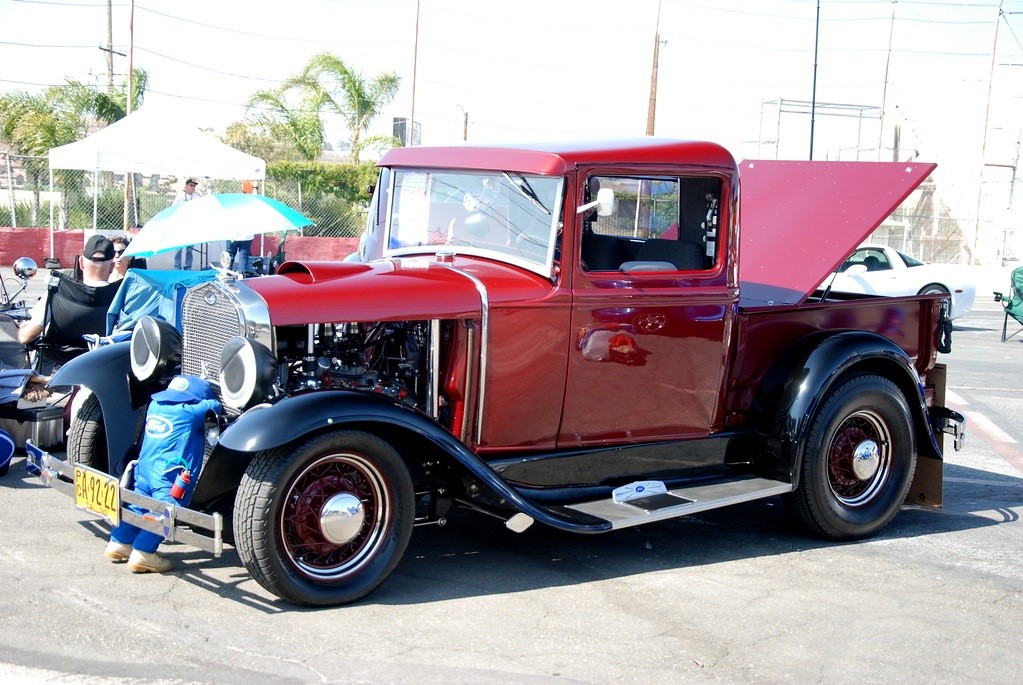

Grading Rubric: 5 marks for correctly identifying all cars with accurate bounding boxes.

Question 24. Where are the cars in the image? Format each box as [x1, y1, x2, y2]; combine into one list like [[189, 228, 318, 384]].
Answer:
[[0, 257, 66, 451], [819, 243, 975, 321], [25, 144, 965, 609]]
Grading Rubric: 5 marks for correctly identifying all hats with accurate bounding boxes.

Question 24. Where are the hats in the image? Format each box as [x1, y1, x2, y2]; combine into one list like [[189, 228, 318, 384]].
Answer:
[[186, 179, 198, 185], [151, 374, 214, 404], [82, 234, 115, 262]]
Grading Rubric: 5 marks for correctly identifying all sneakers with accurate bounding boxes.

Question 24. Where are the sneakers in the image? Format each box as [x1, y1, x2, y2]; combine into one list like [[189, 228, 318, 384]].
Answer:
[[104, 540, 133, 562], [127, 549, 173, 573]]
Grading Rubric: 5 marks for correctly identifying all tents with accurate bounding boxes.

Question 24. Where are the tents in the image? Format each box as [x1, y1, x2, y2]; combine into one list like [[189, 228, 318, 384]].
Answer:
[[49, 99, 266, 264]]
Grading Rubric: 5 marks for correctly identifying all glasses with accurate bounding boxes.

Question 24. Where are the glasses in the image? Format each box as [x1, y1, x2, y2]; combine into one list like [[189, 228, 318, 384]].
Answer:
[[114, 249, 125, 255], [190, 185, 196, 187]]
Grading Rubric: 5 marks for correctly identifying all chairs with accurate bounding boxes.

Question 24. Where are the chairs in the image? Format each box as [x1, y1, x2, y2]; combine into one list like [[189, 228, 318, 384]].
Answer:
[[991, 267, 1023, 341], [863, 255, 880, 271], [20, 255, 147, 407], [580, 234, 625, 272], [635, 239, 704, 269]]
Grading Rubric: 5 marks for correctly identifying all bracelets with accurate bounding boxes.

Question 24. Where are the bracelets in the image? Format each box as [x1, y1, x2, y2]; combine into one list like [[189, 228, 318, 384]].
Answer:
[[253, 187, 257, 189]]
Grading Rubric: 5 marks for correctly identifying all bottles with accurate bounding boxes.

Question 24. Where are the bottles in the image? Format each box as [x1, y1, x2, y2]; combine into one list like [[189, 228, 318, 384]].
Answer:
[[706, 199, 718, 257]]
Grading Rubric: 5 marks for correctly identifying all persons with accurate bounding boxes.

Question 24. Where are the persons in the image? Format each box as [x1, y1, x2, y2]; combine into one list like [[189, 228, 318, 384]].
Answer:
[[171, 178, 201, 269], [225, 181, 258, 272], [18, 235, 133, 375], [105, 375, 223, 573]]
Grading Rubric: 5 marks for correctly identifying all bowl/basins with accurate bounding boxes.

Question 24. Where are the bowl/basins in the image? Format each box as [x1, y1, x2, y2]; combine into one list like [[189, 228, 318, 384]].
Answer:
[[0, 429, 15, 477]]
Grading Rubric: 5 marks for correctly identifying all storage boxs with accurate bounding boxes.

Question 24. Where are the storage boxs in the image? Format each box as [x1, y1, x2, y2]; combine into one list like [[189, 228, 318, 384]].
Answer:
[[0, 402, 67, 454]]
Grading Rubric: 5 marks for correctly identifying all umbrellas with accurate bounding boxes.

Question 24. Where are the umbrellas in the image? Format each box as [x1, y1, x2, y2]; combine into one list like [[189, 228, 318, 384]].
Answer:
[[120, 193, 317, 260]]
